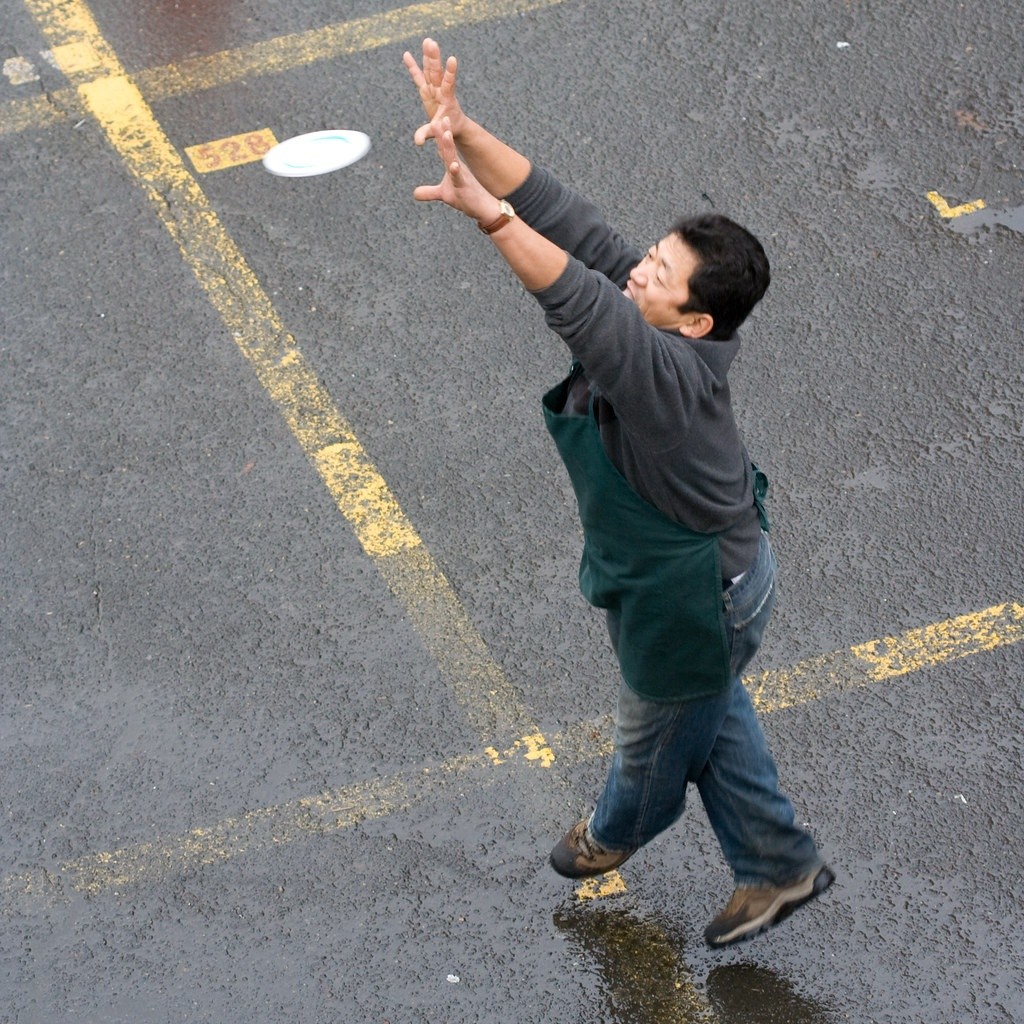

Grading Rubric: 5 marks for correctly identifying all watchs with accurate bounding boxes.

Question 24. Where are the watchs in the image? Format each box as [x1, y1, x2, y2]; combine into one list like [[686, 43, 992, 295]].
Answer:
[[477, 197, 516, 236]]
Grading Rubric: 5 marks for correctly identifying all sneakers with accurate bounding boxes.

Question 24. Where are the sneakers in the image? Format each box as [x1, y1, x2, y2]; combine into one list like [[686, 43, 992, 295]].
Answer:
[[550, 818, 640, 879], [705, 860, 835, 948]]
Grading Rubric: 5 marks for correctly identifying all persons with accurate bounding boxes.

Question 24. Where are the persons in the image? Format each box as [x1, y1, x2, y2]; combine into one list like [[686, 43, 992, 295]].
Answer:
[[398, 36, 842, 948]]
